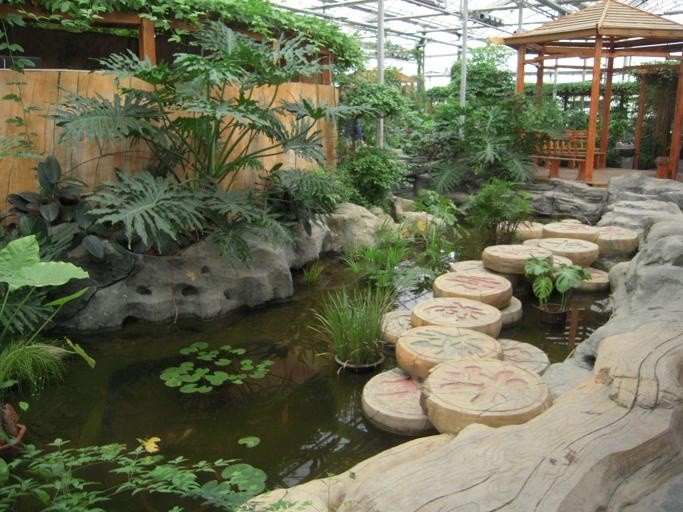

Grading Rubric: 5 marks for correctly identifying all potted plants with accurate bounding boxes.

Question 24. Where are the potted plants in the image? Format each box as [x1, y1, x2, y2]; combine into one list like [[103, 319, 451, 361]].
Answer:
[[309, 275, 399, 376], [1, 234, 97, 463], [526, 251, 592, 325]]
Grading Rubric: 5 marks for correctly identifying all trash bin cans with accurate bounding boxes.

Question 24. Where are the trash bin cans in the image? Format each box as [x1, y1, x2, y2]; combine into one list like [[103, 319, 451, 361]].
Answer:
[[614, 140, 636, 169]]
